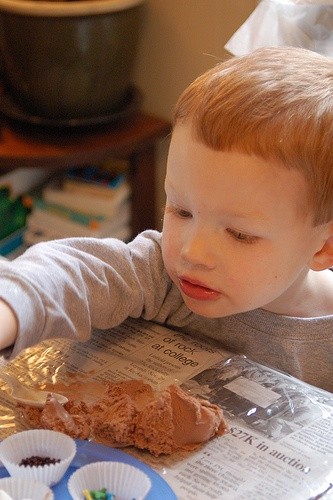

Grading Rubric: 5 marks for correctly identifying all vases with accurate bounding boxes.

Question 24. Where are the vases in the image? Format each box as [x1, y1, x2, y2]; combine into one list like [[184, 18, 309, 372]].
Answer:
[[0, 1, 145, 140]]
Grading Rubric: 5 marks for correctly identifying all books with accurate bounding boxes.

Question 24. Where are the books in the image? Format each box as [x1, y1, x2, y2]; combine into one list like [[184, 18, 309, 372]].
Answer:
[[23, 177, 134, 249]]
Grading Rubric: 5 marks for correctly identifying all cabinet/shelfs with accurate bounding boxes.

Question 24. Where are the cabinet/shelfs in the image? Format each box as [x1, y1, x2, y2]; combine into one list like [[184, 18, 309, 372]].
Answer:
[[1, 118, 172, 237]]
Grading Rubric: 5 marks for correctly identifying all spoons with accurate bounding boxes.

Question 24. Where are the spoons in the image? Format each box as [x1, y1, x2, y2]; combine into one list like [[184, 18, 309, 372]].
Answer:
[[0, 367, 69, 408]]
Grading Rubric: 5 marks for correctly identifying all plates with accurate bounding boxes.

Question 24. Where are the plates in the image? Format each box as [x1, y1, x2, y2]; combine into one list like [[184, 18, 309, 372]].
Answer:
[[0, 439, 179, 500]]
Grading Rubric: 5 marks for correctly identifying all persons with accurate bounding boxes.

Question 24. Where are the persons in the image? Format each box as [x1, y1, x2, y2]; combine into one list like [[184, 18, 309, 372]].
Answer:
[[0, 45, 333, 393]]
